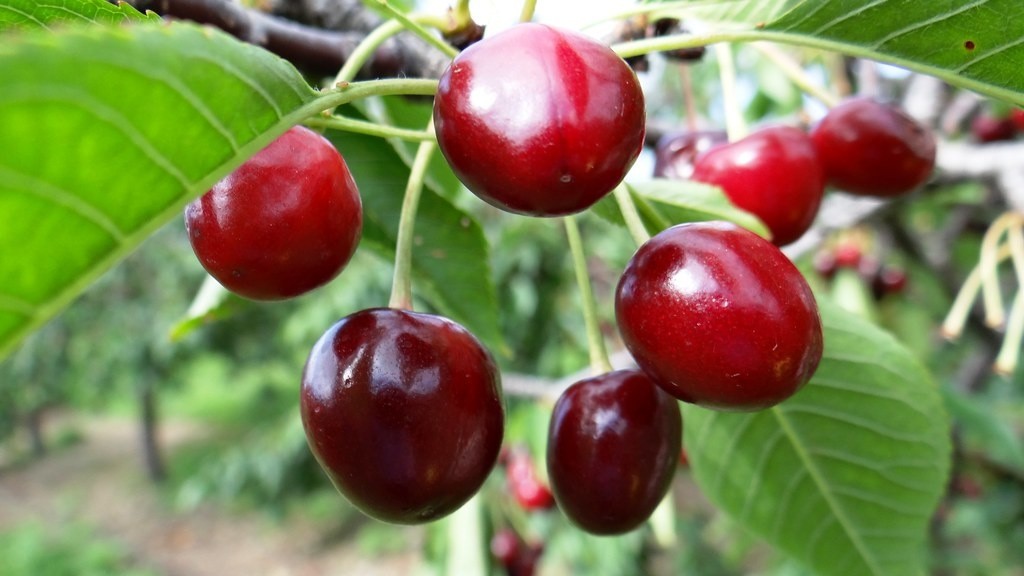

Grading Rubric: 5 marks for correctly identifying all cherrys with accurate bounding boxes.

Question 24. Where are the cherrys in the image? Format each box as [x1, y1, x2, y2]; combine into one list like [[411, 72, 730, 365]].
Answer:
[[181, 22, 1024, 576]]
